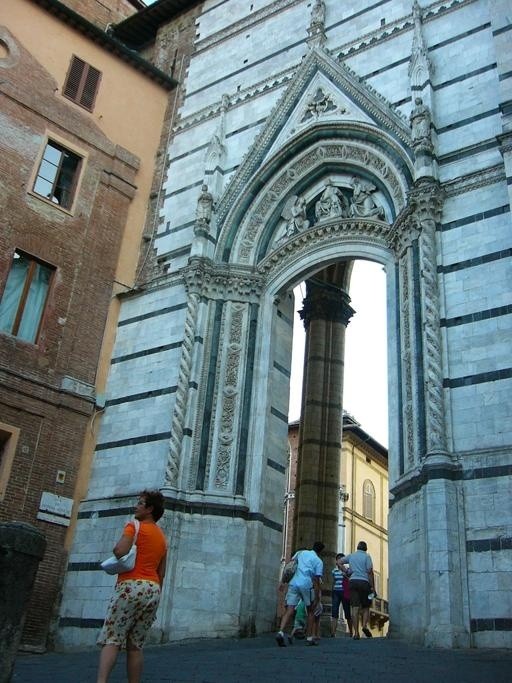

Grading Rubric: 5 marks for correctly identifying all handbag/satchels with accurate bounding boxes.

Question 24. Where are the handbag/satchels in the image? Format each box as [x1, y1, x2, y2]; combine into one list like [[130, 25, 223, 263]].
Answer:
[[282, 560, 297, 584], [100, 544, 137, 575]]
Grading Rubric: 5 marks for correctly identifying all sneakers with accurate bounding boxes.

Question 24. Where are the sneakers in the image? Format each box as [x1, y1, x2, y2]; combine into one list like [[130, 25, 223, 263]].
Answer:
[[306, 640, 319, 646], [354, 627, 372, 640], [275, 630, 287, 647], [287, 635, 294, 645], [331, 632, 353, 638]]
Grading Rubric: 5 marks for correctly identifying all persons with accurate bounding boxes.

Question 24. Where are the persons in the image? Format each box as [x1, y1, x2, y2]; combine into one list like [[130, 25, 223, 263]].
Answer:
[[327, 552, 354, 638], [314, 179, 343, 222], [285, 578, 322, 645], [346, 175, 385, 218], [286, 195, 311, 235], [272, 540, 325, 647], [95, 488, 170, 683], [335, 540, 377, 640]]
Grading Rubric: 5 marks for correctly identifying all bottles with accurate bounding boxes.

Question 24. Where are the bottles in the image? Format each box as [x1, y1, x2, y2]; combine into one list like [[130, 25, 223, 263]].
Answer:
[[368, 592, 375, 599]]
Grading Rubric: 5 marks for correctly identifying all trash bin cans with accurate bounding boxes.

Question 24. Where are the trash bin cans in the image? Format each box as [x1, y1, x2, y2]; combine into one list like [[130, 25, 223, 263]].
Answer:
[[0, 520, 47, 681]]
